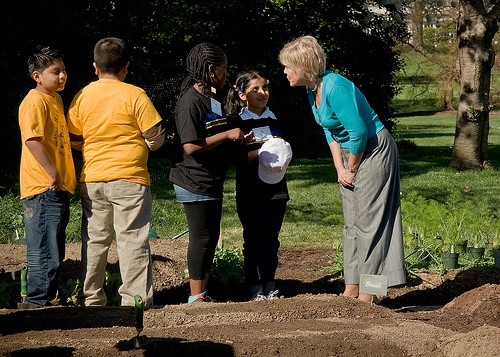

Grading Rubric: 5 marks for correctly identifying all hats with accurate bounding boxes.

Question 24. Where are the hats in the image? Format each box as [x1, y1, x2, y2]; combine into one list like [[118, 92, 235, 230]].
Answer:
[[258, 138, 292, 185]]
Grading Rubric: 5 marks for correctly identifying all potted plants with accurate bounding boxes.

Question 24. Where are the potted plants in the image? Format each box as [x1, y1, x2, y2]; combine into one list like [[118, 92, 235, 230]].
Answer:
[[400, 191, 500, 269]]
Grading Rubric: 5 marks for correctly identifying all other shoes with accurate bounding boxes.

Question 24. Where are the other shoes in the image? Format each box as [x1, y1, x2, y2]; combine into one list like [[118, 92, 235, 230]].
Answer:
[[247, 292, 266, 302], [267, 289, 281, 300], [188, 291, 208, 304]]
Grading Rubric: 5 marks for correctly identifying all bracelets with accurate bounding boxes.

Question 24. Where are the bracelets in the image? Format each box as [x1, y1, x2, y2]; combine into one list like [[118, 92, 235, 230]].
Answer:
[[346, 167, 355, 173]]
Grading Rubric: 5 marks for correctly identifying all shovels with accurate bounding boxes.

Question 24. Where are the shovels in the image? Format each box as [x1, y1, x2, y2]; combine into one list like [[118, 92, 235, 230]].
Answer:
[[133, 295, 148, 349]]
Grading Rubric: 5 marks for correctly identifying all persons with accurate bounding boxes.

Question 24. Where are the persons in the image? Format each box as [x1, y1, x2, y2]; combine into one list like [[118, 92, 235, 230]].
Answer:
[[221, 70, 290, 302], [66, 37, 166, 308], [167, 42, 254, 306], [17, 45, 85, 309], [280, 35, 408, 306]]
